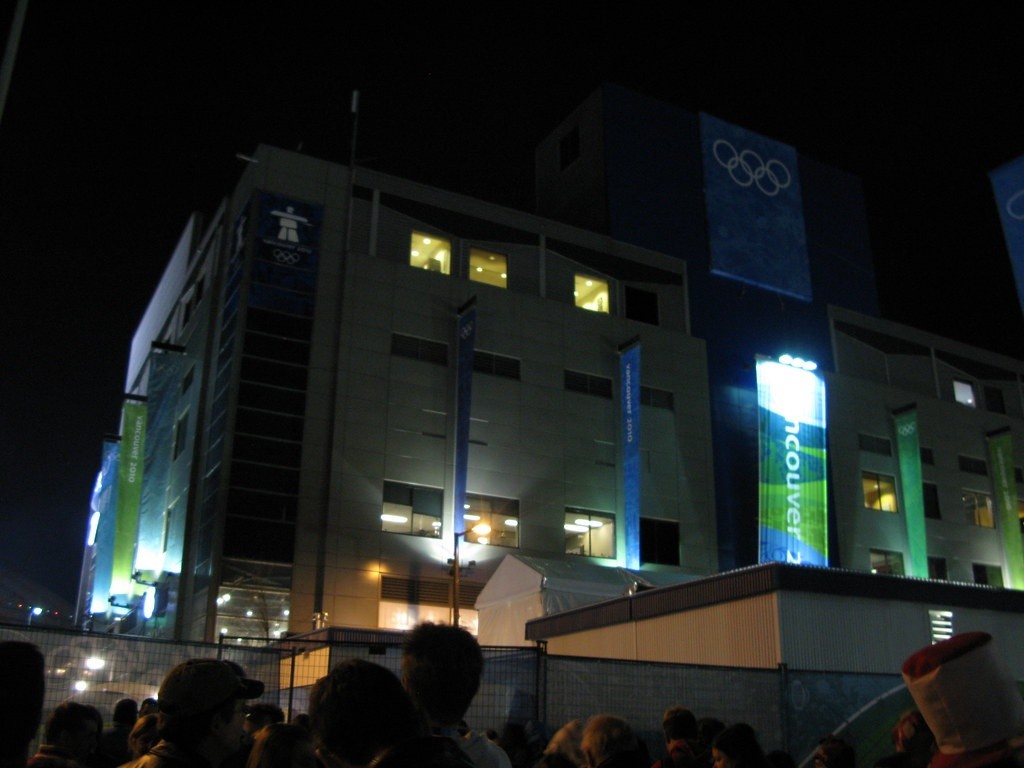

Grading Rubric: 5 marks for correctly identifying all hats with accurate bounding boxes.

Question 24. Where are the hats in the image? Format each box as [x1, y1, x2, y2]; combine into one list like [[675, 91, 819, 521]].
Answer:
[[902, 631, 1024, 754], [157, 657, 265, 735]]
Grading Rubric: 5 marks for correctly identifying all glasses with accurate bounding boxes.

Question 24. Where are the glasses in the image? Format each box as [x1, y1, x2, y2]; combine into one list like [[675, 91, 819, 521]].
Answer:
[[230, 704, 255, 718]]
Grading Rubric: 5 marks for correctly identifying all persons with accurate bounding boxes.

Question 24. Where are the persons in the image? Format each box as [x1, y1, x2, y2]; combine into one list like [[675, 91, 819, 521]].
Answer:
[[0, 621, 1024, 768]]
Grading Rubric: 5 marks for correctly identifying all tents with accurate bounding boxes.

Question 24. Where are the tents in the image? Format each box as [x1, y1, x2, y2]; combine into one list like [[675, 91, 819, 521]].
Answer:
[[475, 555, 707, 647]]
